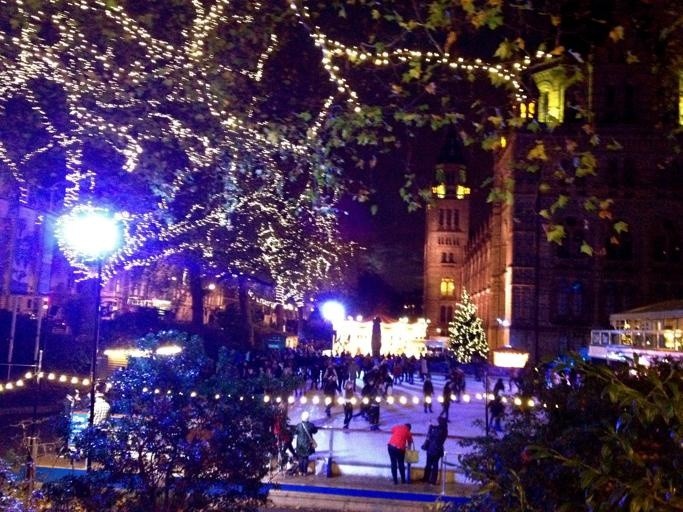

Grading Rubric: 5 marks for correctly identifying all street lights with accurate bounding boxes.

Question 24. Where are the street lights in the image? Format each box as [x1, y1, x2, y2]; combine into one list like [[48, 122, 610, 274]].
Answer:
[[321, 300, 344, 358], [484, 343, 529, 452], [59, 207, 120, 498]]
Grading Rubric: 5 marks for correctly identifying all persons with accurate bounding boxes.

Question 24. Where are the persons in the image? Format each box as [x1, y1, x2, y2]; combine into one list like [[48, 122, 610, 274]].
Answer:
[[291, 411, 317, 476], [420, 416, 448, 485], [386, 422, 413, 484], [262, 344, 507, 433]]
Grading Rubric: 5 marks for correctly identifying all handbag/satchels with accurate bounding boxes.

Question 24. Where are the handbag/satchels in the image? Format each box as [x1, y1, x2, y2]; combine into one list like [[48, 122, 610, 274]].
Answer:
[[310, 438, 318, 449], [420, 439, 430, 450], [404, 441, 418, 462]]
